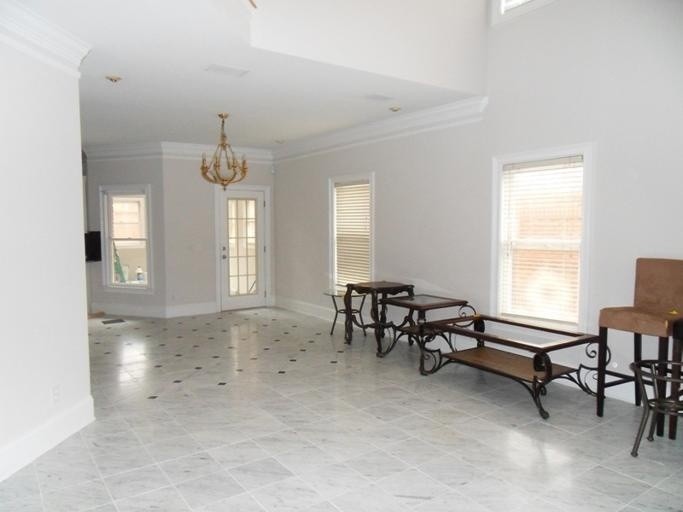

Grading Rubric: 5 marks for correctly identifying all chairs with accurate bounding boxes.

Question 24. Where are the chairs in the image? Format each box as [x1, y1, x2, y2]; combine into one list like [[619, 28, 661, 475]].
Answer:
[[629, 360, 683, 457], [596, 257, 682, 436]]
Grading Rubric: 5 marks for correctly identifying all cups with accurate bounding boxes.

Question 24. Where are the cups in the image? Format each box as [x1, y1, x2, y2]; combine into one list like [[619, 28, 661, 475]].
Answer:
[[121, 264, 129, 280]]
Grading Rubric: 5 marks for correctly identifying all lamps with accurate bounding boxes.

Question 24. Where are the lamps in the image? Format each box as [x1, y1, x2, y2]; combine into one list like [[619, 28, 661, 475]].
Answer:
[[200, 113, 248, 190]]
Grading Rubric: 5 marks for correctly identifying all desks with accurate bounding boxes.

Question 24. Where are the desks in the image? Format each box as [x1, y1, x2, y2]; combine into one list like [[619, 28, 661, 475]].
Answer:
[[416, 314, 611, 419], [324, 290, 367, 336], [344, 280, 416, 352], [370, 294, 477, 374]]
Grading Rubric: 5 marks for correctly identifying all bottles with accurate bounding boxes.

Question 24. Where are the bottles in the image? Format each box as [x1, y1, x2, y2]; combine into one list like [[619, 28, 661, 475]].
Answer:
[[135, 265, 142, 282]]
